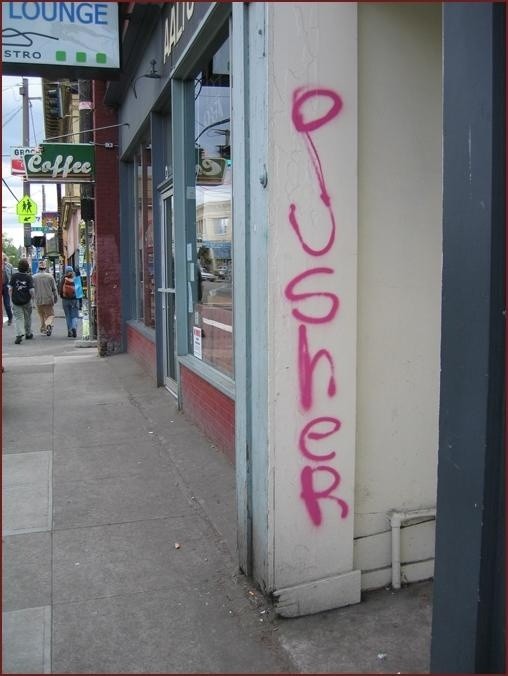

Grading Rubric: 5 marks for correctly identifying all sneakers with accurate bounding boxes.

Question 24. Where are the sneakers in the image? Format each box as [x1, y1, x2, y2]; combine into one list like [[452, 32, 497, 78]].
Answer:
[[68, 328, 77, 337], [14, 335, 22, 344], [25, 333, 33, 339], [46, 324, 52, 336], [7, 316, 12, 325]]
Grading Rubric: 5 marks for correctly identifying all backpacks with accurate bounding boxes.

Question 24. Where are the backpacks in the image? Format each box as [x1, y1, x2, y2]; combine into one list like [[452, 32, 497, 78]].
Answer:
[[62, 275, 75, 298]]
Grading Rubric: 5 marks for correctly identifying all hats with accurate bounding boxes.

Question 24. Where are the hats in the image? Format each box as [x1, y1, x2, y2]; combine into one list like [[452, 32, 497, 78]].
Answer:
[[64, 265, 73, 272], [38, 260, 46, 268]]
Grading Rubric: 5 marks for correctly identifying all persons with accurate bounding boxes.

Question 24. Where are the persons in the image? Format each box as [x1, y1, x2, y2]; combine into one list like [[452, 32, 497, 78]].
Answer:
[[197, 245, 216, 337], [2, 252, 84, 344]]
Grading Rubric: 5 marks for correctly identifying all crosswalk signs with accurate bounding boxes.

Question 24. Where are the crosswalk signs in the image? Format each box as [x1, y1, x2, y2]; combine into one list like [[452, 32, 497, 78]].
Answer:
[[16, 195, 38, 214]]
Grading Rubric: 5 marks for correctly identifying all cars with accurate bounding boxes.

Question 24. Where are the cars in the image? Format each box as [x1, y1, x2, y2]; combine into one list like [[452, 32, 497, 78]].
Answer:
[[199, 268, 216, 282]]
